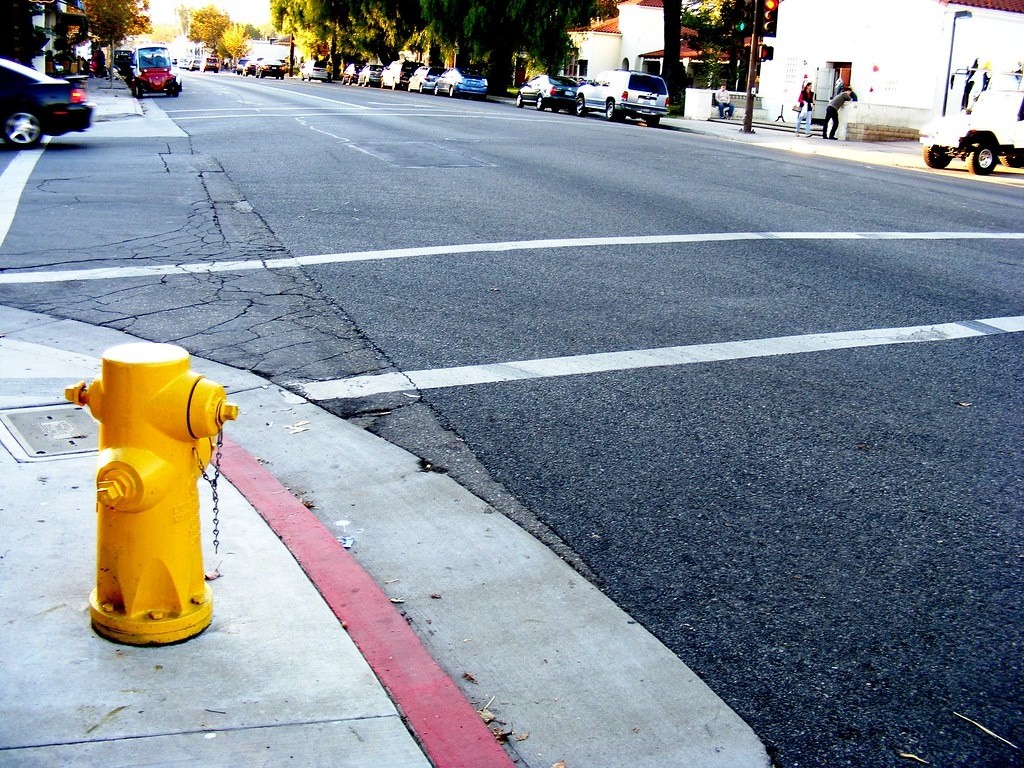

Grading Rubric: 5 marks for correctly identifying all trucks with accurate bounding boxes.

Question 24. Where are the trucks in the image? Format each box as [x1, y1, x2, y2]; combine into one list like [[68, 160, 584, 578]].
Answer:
[[917, 66, 1024, 176]]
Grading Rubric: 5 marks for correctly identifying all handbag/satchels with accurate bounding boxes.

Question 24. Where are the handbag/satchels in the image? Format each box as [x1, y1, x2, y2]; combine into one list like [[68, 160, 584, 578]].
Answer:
[[800, 103, 808, 118], [792, 105, 802, 112]]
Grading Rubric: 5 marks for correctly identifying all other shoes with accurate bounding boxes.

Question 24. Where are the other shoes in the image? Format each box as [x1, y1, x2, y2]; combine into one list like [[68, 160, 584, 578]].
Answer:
[[805, 134, 813, 138], [823, 137, 829, 139], [795, 133, 799, 137], [829, 137, 838, 140]]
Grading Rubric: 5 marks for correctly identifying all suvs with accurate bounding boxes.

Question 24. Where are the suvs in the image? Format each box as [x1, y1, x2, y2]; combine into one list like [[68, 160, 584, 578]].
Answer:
[[379, 60, 425, 91], [575, 68, 670, 126]]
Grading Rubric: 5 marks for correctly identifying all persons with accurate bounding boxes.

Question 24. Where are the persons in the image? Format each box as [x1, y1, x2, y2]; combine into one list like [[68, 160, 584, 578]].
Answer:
[[326, 61, 333, 83], [795, 82, 816, 137], [823, 86, 858, 140], [301, 63, 305, 69], [715, 84, 735, 120]]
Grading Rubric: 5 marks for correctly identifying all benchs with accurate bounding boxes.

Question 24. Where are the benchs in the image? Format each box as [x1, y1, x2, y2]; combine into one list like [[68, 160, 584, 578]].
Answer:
[[712, 92, 765, 117]]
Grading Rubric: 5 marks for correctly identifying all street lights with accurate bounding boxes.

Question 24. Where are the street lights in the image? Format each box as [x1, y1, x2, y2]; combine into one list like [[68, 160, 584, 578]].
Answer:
[[940, 9, 972, 117]]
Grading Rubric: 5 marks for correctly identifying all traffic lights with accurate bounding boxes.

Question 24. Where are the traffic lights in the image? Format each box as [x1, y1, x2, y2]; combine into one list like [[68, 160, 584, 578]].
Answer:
[[757, 0, 779, 39], [759, 45, 774, 61], [733, 0, 756, 38]]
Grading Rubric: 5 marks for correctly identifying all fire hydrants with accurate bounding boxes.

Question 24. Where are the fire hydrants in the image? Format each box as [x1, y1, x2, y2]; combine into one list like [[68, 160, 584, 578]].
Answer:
[[64, 341, 241, 649]]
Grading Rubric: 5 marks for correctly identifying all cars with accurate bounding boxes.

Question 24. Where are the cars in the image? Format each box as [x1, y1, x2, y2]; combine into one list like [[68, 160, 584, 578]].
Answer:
[[434, 67, 489, 102], [0, 56, 95, 152], [356, 64, 386, 88], [114, 44, 221, 99], [235, 57, 289, 80], [407, 66, 447, 94], [300, 59, 333, 83], [515, 75, 580, 113], [341, 63, 366, 86]]
